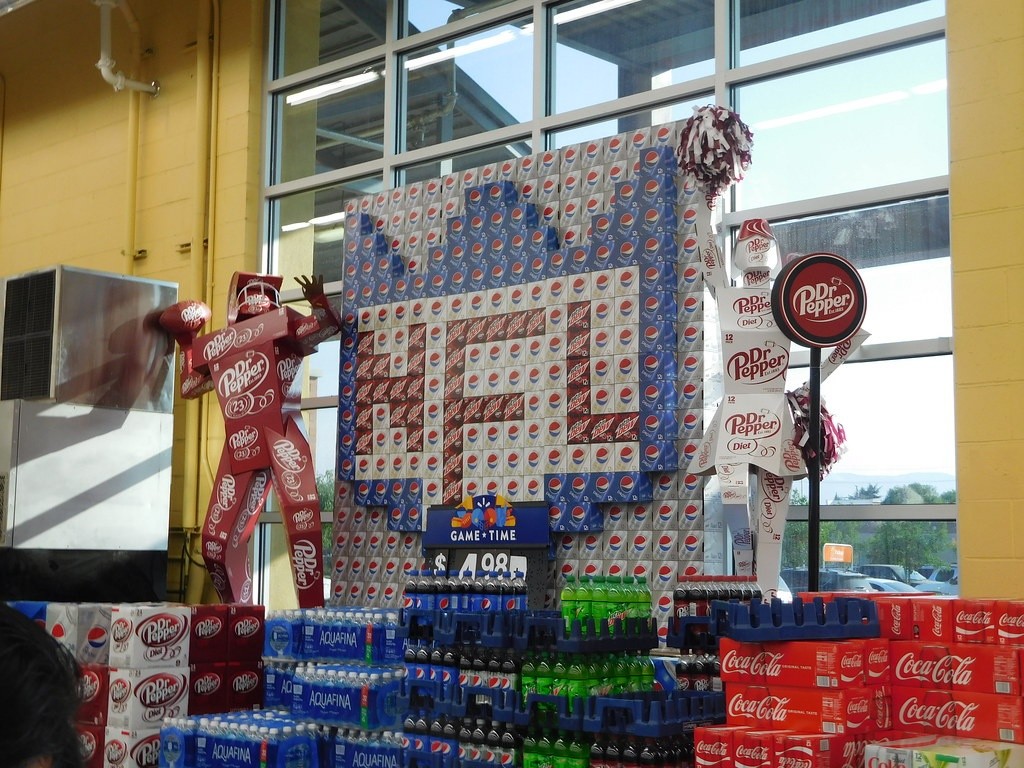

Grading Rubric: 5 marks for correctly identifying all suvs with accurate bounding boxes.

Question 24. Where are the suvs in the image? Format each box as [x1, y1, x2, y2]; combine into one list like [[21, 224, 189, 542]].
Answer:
[[779, 567, 876, 598], [776, 574, 793, 605]]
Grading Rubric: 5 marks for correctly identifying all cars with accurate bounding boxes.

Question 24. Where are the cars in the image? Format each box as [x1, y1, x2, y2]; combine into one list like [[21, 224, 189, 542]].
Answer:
[[862, 565, 961, 597], [868, 577, 920, 595]]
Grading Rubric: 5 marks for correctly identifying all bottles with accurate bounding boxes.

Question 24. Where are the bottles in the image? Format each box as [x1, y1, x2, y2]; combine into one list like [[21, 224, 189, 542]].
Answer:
[[165, 558, 762, 768]]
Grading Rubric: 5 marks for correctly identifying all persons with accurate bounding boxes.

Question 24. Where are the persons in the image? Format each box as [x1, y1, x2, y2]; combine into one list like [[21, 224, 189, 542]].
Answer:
[[2, 604, 91, 768]]
